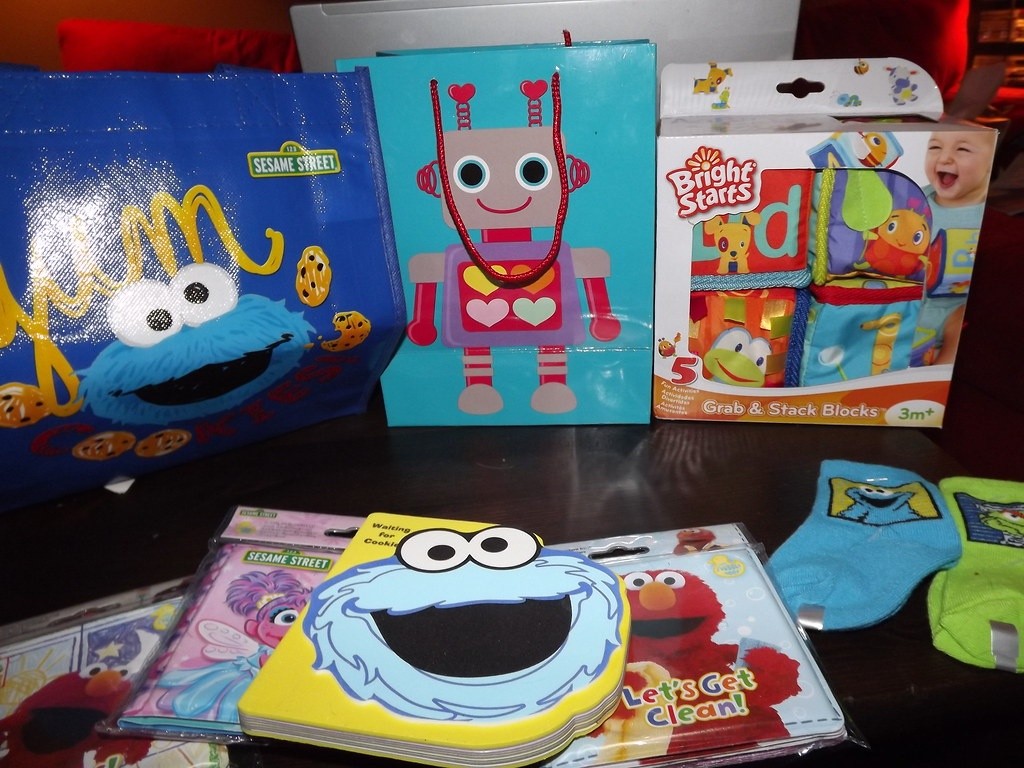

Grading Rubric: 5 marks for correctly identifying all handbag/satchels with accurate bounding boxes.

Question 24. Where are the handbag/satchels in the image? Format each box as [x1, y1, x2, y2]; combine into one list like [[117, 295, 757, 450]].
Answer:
[[0, 63, 411, 513], [328, 37, 654, 428]]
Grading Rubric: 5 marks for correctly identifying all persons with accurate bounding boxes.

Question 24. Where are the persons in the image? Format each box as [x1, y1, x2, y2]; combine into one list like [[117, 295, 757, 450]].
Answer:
[[903, 132, 998, 365]]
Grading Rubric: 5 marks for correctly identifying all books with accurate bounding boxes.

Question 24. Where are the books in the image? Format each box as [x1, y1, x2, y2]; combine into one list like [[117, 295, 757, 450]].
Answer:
[[236, 511, 632, 766]]
